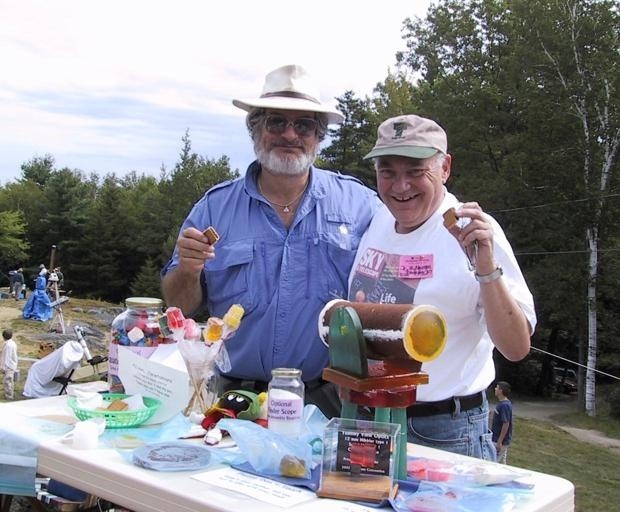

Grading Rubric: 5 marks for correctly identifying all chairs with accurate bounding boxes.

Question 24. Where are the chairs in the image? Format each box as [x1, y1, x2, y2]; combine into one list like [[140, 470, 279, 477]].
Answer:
[[27, 478, 112, 511]]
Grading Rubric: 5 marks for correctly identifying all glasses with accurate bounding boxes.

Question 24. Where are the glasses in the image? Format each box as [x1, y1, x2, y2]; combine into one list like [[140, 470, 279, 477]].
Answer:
[[264, 114, 319, 137]]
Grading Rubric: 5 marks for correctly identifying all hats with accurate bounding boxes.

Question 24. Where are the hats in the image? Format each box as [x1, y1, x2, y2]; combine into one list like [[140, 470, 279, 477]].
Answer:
[[17, 268, 23, 272], [39, 264, 44, 268], [233, 64, 346, 124], [363, 115, 447, 160]]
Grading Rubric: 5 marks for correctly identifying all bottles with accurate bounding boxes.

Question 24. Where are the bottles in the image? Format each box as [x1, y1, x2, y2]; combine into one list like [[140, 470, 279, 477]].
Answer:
[[267, 367, 305, 440], [109, 297, 166, 393]]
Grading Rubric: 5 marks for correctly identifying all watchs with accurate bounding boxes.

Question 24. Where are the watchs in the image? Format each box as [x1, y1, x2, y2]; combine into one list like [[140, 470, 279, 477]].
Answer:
[[475, 265, 505, 282]]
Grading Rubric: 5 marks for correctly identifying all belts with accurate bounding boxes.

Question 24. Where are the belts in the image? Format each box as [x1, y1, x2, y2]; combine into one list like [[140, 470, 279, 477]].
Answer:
[[219, 375, 327, 393], [357, 392, 484, 418]]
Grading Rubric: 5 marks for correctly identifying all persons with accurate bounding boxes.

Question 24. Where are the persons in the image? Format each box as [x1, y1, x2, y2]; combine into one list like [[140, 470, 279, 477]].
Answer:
[[22, 268, 54, 323], [492, 381, 512, 466], [349, 115, 536, 459], [159, 64, 383, 420], [15, 268, 25, 301], [0, 330, 17, 399]]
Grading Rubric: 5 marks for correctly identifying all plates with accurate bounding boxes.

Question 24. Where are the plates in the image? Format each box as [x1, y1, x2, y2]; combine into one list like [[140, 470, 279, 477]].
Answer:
[[70, 393, 162, 430]]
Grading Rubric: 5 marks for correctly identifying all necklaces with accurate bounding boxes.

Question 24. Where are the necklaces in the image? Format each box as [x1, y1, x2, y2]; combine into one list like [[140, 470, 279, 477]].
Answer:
[[257, 174, 309, 213]]
